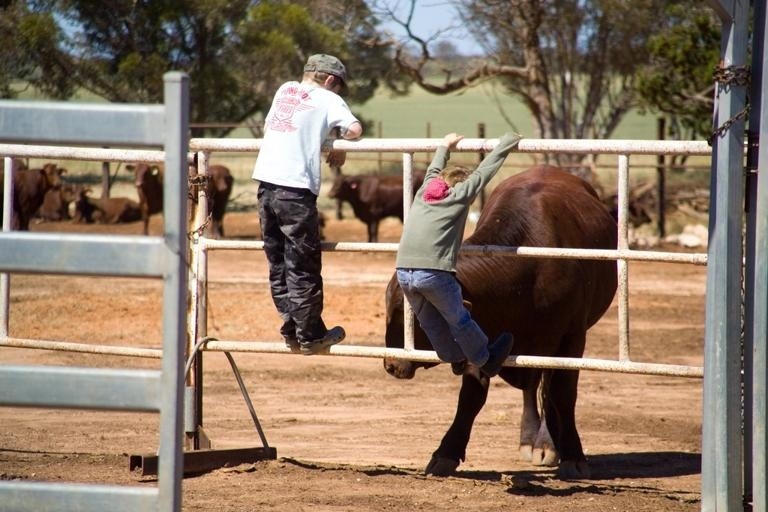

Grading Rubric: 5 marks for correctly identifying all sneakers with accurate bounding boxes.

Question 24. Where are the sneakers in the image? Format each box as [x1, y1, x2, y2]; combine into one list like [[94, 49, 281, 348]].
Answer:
[[479, 332, 514, 377], [451, 360, 466, 375]]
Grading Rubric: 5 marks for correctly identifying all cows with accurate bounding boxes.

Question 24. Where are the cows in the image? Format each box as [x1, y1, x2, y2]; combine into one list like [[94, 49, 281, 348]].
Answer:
[[382, 163, 619, 479], [202, 163, 233, 240], [0, 156, 164, 236], [327, 168, 427, 243]]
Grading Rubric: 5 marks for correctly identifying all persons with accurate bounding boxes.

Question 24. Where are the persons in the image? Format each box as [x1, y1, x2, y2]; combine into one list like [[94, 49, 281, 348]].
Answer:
[[250, 53, 364, 356], [395, 131, 524, 380]]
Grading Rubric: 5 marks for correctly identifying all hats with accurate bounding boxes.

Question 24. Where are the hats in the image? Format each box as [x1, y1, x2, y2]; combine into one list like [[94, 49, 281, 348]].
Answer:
[[303, 54, 349, 97]]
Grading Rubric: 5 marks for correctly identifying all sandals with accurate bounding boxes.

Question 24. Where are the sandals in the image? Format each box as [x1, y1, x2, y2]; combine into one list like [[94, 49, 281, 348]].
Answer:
[[289, 326, 346, 355]]
